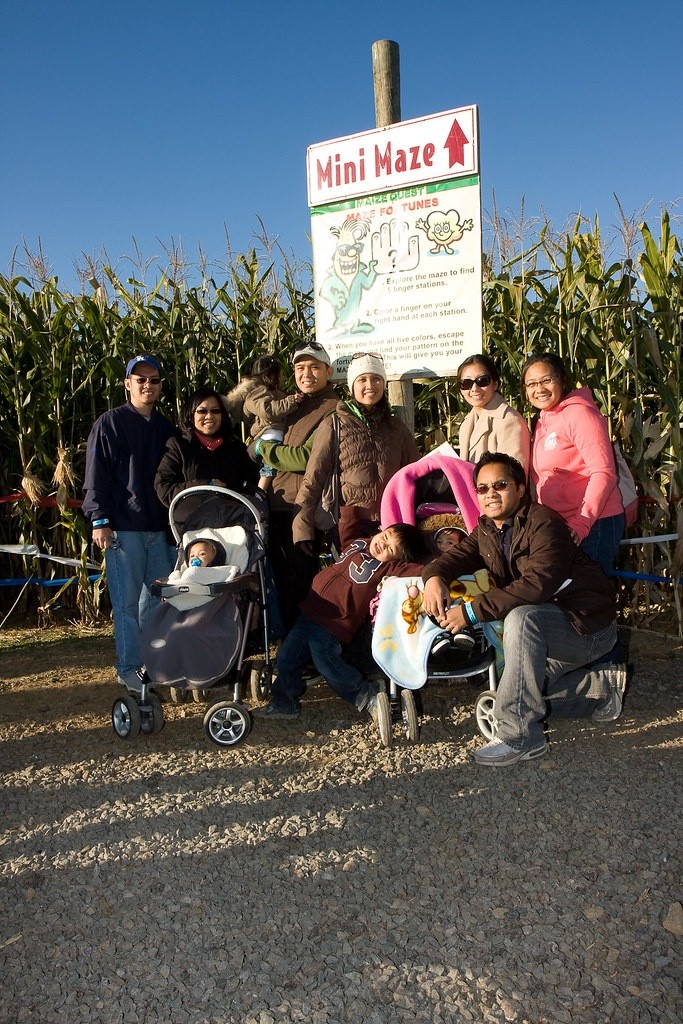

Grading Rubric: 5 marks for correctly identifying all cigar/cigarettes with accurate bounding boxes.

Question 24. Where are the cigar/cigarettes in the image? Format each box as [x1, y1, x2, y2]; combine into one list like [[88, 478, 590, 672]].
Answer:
[[101, 547, 105, 552]]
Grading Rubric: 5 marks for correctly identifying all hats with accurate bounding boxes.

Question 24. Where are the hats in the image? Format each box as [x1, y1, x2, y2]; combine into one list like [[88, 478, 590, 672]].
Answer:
[[347, 355, 387, 397], [292, 343, 331, 367], [126, 355, 162, 379]]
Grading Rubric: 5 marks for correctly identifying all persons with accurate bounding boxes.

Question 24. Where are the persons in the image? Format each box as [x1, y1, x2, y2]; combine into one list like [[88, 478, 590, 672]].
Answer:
[[417, 513, 476, 658], [141, 538, 243, 687], [422, 450, 626, 768], [249, 523, 467, 721], [245, 342, 342, 643], [456, 354, 529, 493], [221, 355, 303, 502], [292, 352, 419, 561], [154, 389, 253, 541], [80, 355, 172, 693], [519, 352, 626, 667]]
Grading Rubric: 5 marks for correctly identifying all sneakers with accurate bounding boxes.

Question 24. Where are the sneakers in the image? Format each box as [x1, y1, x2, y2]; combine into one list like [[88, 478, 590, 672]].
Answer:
[[453, 627, 475, 651], [252, 704, 301, 720], [473, 735, 549, 766], [590, 662, 626, 722], [354, 680, 387, 725], [117, 668, 145, 693], [431, 633, 450, 656]]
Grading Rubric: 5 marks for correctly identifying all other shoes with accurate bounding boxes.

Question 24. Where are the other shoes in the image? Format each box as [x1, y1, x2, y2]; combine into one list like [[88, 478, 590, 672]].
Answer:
[[256, 487, 267, 502], [301, 669, 323, 686]]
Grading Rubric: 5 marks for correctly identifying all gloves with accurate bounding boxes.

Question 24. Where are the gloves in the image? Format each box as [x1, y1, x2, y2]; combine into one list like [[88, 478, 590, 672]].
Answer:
[[299, 540, 314, 558], [246, 438, 265, 464]]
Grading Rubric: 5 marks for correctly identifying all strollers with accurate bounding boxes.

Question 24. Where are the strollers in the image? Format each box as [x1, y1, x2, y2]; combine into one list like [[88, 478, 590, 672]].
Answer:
[[112, 483, 290, 746], [376, 455, 506, 747]]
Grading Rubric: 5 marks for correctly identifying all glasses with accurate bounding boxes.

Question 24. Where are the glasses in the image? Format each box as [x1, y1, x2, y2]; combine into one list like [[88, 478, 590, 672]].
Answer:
[[458, 374, 494, 390], [350, 352, 384, 366], [294, 341, 329, 360], [130, 378, 161, 384], [194, 409, 222, 414], [523, 375, 559, 388], [475, 480, 516, 494]]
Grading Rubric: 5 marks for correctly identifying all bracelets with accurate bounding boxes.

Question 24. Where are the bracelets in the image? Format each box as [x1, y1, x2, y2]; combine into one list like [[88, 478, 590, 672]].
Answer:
[[91, 519, 109, 527], [254, 438, 263, 455], [464, 601, 478, 625]]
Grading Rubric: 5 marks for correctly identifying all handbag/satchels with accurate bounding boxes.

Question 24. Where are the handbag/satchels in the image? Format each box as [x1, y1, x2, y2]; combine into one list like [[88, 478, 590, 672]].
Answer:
[[612, 440, 638, 527]]
